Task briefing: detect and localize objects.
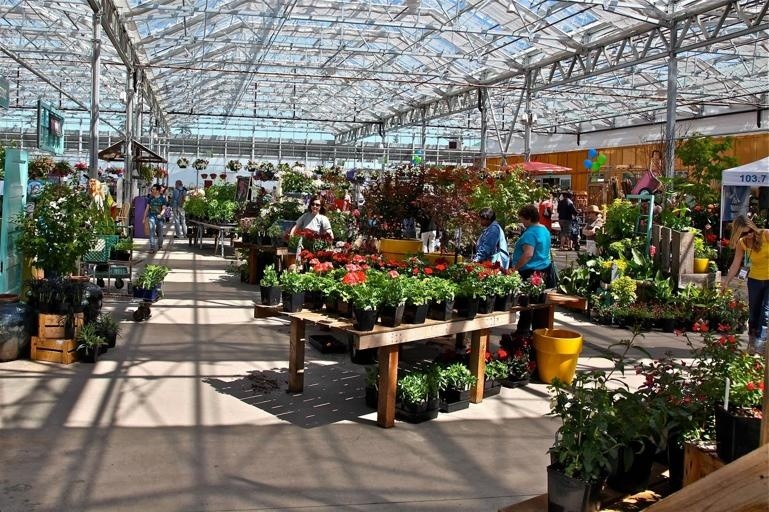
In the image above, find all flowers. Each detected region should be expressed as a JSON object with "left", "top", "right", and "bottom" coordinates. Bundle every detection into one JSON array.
[
  {"left": 261, "top": 250, "right": 547, "bottom": 285},
  {"left": 634, "top": 317, "right": 768, "bottom": 506},
  {"left": 496, "top": 338, "right": 537, "bottom": 380}
]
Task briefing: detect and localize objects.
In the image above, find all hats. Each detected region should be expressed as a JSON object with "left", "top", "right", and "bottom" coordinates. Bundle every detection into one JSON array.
[{"left": 585, "top": 205, "right": 601, "bottom": 215}]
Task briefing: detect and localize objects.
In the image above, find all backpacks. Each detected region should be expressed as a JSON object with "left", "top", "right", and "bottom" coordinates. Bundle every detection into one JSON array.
[{"left": 175, "top": 187, "right": 188, "bottom": 207}]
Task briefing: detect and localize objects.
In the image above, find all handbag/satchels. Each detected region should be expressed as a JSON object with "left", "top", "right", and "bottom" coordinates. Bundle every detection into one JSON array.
[
  {"left": 545, "top": 261, "right": 560, "bottom": 288},
  {"left": 544, "top": 206, "right": 552, "bottom": 218}
]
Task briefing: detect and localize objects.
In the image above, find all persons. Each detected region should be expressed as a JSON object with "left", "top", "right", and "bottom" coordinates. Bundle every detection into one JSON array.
[
  {"left": 511, "top": 204, "right": 552, "bottom": 337},
  {"left": 288, "top": 194, "right": 335, "bottom": 273},
  {"left": 719, "top": 215, "right": 769, "bottom": 355},
  {"left": 538, "top": 190, "right": 581, "bottom": 251},
  {"left": 582, "top": 205, "right": 603, "bottom": 257},
  {"left": 421, "top": 218, "right": 437, "bottom": 253},
  {"left": 473, "top": 207, "right": 510, "bottom": 270},
  {"left": 142, "top": 180, "right": 187, "bottom": 254},
  {"left": 342, "top": 188, "right": 417, "bottom": 248}
]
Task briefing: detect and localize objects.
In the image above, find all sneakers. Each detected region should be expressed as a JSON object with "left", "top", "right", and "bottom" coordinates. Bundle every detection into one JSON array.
[
  {"left": 149, "top": 246, "right": 164, "bottom": 254},
  {"left": 558, "top": 245, "right": 573, "bottom": 252},
  {"left": 173, "top": 234, "right": 187, "bottom": 240}
]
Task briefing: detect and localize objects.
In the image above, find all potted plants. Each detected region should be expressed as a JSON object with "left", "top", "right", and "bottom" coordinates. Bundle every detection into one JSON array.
[
  {"left": 363, "top": 365, "right": 379, "bottom": 409},
  {"left": 397, "top": 368, "right": 431, "bottom": 414},
  {"left": 8, "top": 155, "right": 166, "bottom": 359},
  {"left": 236, "top": 244, "right": 249, "bottom": 284},
  {"left": 492, "top": 359, "right": 510, "bottom": 387},
  {"left": 422, "top": 369, "right": 450, "bottom": 410},
  {"left": 443, "top": 361, "right": 479, "bottom": 401},
  {"left": 263, "top": 285, "right": 545, "bottom": 333},
  {"left": 484, "top": 361, "right": 498, "bottom": 390},
  {"left": 561, "top": 195, "right": 748, "bottom": 331},
  {"left": 177, "top": 152, "right": 548, "bottom": 236},
  {"left": 543, "top": 330, "right": 668, "bottom": 512}
]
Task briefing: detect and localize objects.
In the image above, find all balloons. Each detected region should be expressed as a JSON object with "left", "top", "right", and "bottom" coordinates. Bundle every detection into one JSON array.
[
  {"left": 591, "top": 161, "right": 600, "bottom": 172},
  {"left": 584, "top": 159, "right": 593, "bottom": 169},
  {"left": 597, "top": 154, "right": 607, "bottom": 165},
  {"left": 410, "top": 150, "right": 422, "bottom": 165},
  {"left": 588, "top": 148, "right": 597, "bottom": 158}
]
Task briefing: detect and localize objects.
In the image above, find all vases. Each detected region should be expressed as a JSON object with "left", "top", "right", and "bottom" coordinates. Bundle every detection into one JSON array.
[{"left": 501, "top": 376, "right": 530, "bottom": 389}]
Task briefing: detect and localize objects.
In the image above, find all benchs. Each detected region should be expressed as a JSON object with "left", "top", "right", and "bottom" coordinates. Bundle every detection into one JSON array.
[{"left": 275, "top": 300, "right": 537, "bottom": 394}]
